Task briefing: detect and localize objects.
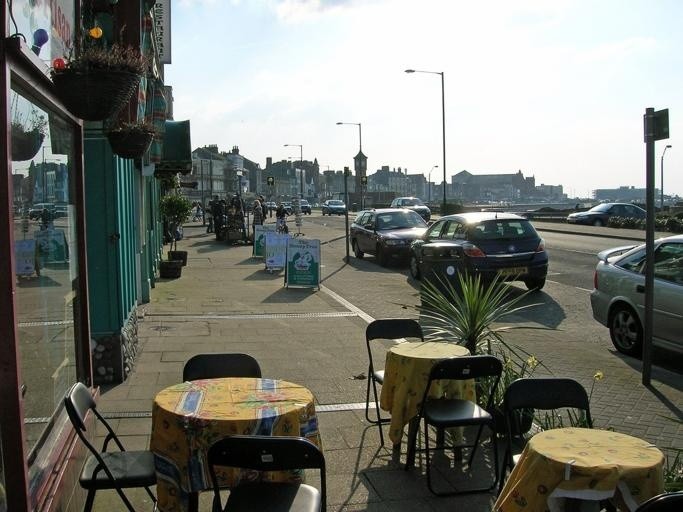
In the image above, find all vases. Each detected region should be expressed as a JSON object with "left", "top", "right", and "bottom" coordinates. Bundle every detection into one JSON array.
[{"left": 496, "top": 401, "right": 536, "bottom": 433}]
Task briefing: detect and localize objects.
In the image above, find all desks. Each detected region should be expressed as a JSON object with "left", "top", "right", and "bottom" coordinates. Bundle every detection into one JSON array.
[
  {"left": 150, "top": 376, "right": 323, "bottom": 511},
  {"left": 490, "top": 427, "right": 667, "bottom": 512},
  {"left": 380, "top": 341, "right": 478, "bottom": 471}
]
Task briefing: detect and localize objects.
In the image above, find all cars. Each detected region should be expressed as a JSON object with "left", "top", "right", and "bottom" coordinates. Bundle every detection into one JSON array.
[
  {"left": 588, "top": 233, "right": 683, "bottom": 357},
  {"left": 247, "top": 198, "right": 312, "bottom": 215},
  {"left": 347, "top": 195, "right": 550, "bottom": 295},
  {"left": 320, "top": 198, "right": 348, "bottom": 216},
  {"left": 565, "top": 202, "right": 647, "bottom": 227},
  {"left": 13, "top": 202, "right": 71, "bottom": 221}
]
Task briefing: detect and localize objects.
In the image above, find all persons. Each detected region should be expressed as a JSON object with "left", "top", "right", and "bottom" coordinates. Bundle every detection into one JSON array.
[
  {"left": 275, "top": 205, "right": 289, "bottom": 226},
  {"left": 191, "top": 193, "right": 266, "bottom": 237}
]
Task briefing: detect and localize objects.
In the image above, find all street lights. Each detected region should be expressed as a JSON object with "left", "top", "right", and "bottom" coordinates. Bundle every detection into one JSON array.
[
  {"left": 335, "top": 121, "right": 363, "bottom": 209},
  {"left": 15, "top": 145, "right": 61, "bottom": 202},
  {"left": 403, "top": 67, "right": 448, "bottom": 216},
  {"left": 428, "top": 165, "right": 438, "bottom": 206},
  {"left": 236, "top": 169, "right": 243, "bottom": 196},
  {"left": 288, "top": 156, "right": 301, "bottom": 159},
  {"left": 282, "top": 144, "right": 304, "bottom": 198},
  {"left": 659, "top": 144, "right": 673, "bottom": 218}
]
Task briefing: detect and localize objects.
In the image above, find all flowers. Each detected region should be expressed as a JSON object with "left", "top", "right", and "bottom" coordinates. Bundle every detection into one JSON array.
[{"left": 491, "top": 355, "right": 541, "bottom": 410}]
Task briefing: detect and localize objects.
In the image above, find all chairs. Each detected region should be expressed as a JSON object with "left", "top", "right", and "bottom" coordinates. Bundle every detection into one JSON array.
[
  {"left": 363, "top": 318, "right": 427, "bottom": 445},
  {"left": 403, "top": 355, "right": 504, "bottom": 498},
  {"left": 63, "top": 381, "right": 158, "bottom": 511},
  {"left": 205, "top": 435, "right": 327, "bottom": 511},
  {"left": 497, "top": 378, "right": 594, "bottom": 499},
  {"left": 634, "top": 489, "right": 683, "bottom": 512},
  {"left": 181, "top": 353, "right": 262, "bottom": 383}
]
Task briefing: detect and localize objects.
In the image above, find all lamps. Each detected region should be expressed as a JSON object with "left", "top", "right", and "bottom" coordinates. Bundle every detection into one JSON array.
[
  {"left": 31, "top": 29, "right": 49, "bottom": 57},
  {"left": 80, "top": 27, "right": 102, "bottom": 40}
]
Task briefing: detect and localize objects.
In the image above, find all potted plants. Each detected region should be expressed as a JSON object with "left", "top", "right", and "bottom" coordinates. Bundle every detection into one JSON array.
[
  {"left": 50, "top": 42, "right": 149, "bottom": 123},
  {"left": 156, "top": 195, "right": 192, "bottom": 279},
  {"left": 106, "top": 119, "right": 163, "bottom": 160},
  {"left": 10, "top": 92, "right": 46, "bottom": 162},
  {"left": 163, "top": 191, "right": 188, "bottom": 267}
]
